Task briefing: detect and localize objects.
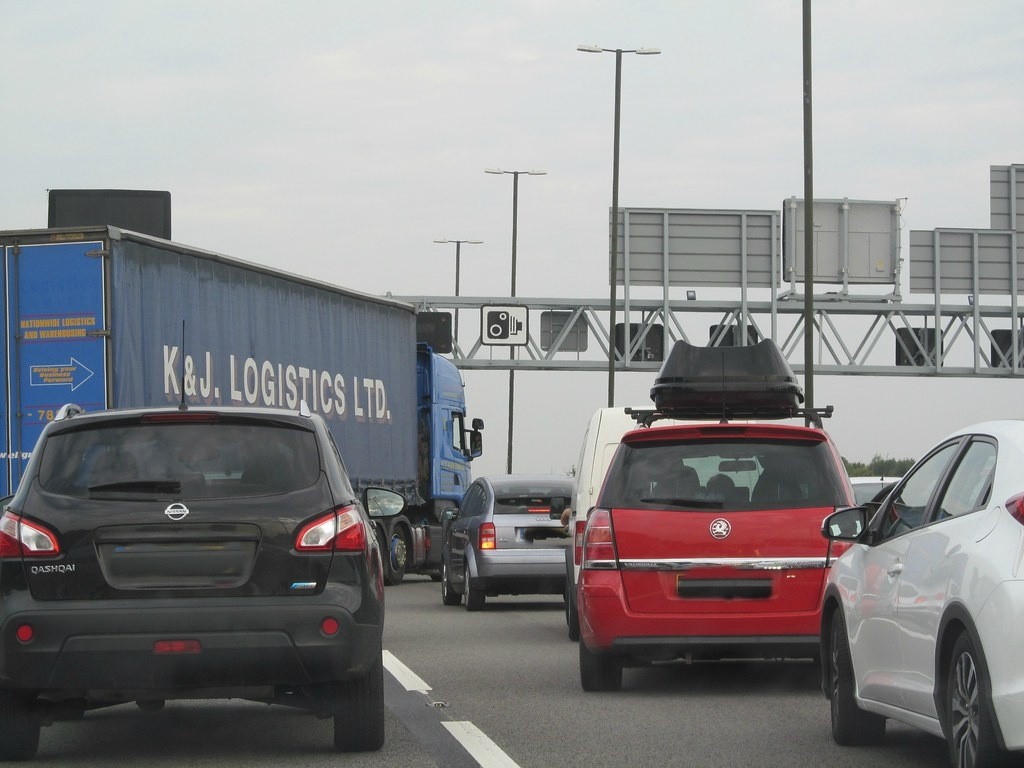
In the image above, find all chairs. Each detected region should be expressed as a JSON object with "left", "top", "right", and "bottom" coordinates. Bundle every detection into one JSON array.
[{"left": 649, "top": 466, "right": 820, "bottom": 505}]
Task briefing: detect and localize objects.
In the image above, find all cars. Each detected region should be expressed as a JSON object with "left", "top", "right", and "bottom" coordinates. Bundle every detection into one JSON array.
[
  {"left": 813, "top": 418, "right": 1024, "bottom": 768},
  {"left": 439, "top": 477, "right": 570, "bottom": 611}
]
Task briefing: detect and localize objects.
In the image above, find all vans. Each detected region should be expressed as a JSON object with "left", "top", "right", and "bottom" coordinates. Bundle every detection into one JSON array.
[{"left": 562, "top": 403, "right": 816, "bottom": 639}]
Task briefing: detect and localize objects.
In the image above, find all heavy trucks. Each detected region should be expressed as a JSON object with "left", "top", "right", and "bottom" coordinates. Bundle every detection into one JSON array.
[{"left": 0, "top": 225, "right": 488, "bottom": 587}]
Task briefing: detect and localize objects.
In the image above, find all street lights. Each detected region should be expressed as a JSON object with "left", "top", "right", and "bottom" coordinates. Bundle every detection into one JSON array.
[
  {"left": 578, "top": 46, "right": 663, "bottom": 409},
  {"left": 483, "top": 167, "right": 550, "bottom": 476},
  {"left": 434, "top": 238, "right": 485, "bottom": 358}
]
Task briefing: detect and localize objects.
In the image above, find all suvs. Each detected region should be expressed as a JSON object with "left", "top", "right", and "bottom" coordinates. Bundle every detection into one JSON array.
[
  {"left": 579, "top": 338, "right": 859, "bottom": 694},
  {"left": 0, "top": 385, "right": 409, "bottom": 760}
]
